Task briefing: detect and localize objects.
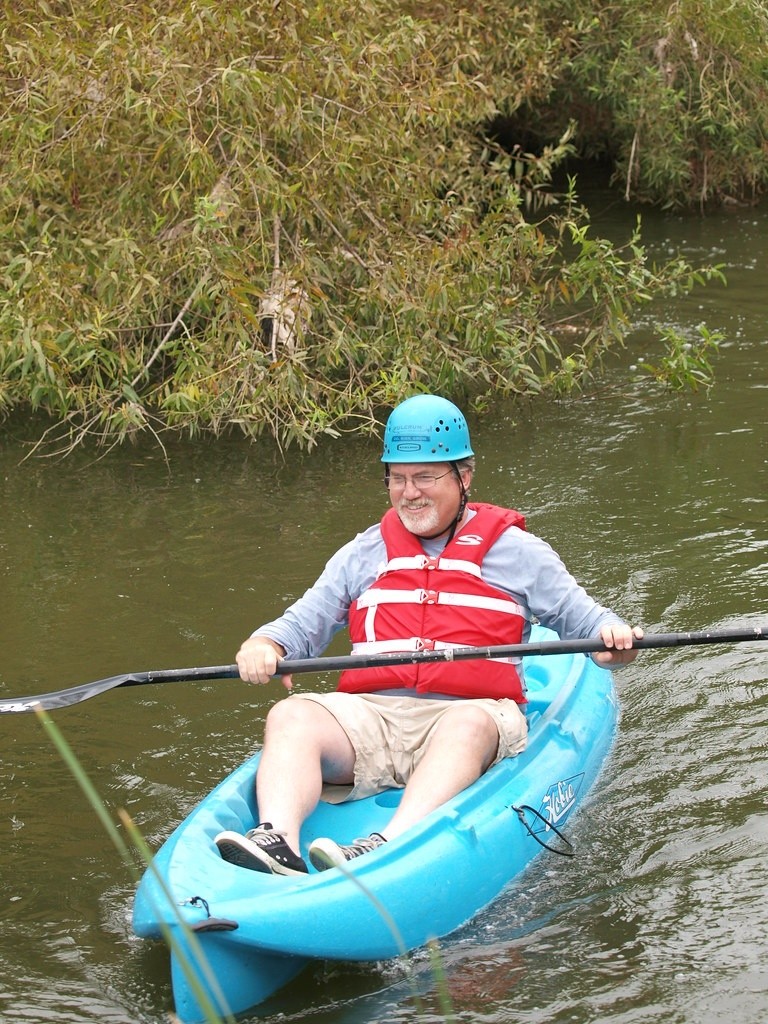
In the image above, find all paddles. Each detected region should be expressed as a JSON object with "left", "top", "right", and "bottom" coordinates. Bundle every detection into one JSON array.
[{"left": 0, "top": 623, "right": 768, "bottom": 714}]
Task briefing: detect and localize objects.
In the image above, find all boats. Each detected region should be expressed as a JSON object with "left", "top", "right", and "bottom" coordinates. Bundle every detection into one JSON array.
[{"left": 130, "top": 622, "right": 622, "bottom": 1024}]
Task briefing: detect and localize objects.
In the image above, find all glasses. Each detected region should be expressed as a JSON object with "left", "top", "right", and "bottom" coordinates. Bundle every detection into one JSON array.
[{"left": 383, "top": 468, "right": 454, "bottom": 489}]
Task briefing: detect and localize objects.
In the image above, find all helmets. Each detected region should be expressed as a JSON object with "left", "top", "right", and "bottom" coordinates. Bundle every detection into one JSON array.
[{"left": 380, "top": 394, "right": 475, "bottom": 462}]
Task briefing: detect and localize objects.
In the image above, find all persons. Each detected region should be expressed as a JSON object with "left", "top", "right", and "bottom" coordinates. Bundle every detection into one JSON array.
[{"left": 214, "top": 395, "right": 644, "bottom": 878}]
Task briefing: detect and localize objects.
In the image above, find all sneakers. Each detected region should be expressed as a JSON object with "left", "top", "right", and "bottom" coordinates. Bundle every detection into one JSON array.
[
  {"left": 213, "top": 823, "right": 308, "bottom": 876},
  {"left": 309, "top": 832, "right": 388, "bottom": 872}
]
